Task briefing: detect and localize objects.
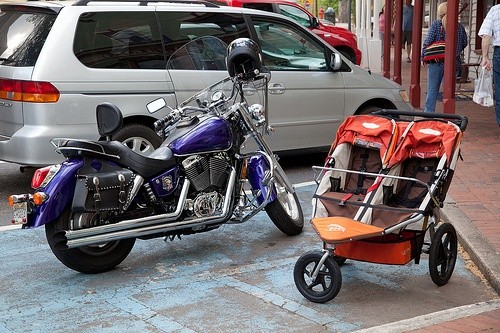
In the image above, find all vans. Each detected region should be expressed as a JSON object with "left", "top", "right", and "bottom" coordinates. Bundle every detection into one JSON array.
[{"left": 0, "top": 0, "right": 415, "bottom": 168}]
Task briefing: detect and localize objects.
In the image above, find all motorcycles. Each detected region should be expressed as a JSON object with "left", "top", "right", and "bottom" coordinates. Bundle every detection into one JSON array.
[{"left": 8, "top": 34, "right": 305, "bottom": 275}]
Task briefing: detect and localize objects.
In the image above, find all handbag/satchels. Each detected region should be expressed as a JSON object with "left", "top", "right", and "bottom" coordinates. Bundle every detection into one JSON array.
[{"left": 423, "top": 20, "right": 446, "bottom": 64}]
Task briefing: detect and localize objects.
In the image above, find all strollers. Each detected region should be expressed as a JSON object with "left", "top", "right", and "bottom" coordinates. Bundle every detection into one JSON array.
[{"left": 293, "top": 109, "right": 468, "bottom": 304}]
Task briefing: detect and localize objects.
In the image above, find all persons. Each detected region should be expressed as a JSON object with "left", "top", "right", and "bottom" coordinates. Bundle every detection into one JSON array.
[
  {"left": 378, "top": 0, "right": 416, "bottom": 62},
  {"left": 421, "top": 2, "right": 467, "bottom": 118},
  {"left": 477, "top": 4, "right": 500, "bottom": 128}
]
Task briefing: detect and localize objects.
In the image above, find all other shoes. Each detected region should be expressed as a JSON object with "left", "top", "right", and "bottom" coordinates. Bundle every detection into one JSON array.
[{"left": 407, "top": 57, "right": 411, "bottom": 63}]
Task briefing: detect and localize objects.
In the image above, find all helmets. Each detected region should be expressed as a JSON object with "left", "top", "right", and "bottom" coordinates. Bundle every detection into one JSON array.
[{"left": 225, "top": 37, "right": 262, "bottom": 80}]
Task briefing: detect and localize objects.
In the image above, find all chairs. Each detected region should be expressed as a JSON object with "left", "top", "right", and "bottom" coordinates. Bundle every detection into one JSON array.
[{"left": 456, "top": 49, "right": 483, "bottom": 87}]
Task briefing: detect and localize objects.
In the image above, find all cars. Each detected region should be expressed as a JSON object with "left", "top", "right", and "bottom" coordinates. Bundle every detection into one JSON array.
[{"left": 207, "top": 0, "right": 362, "bottom": 71}]
